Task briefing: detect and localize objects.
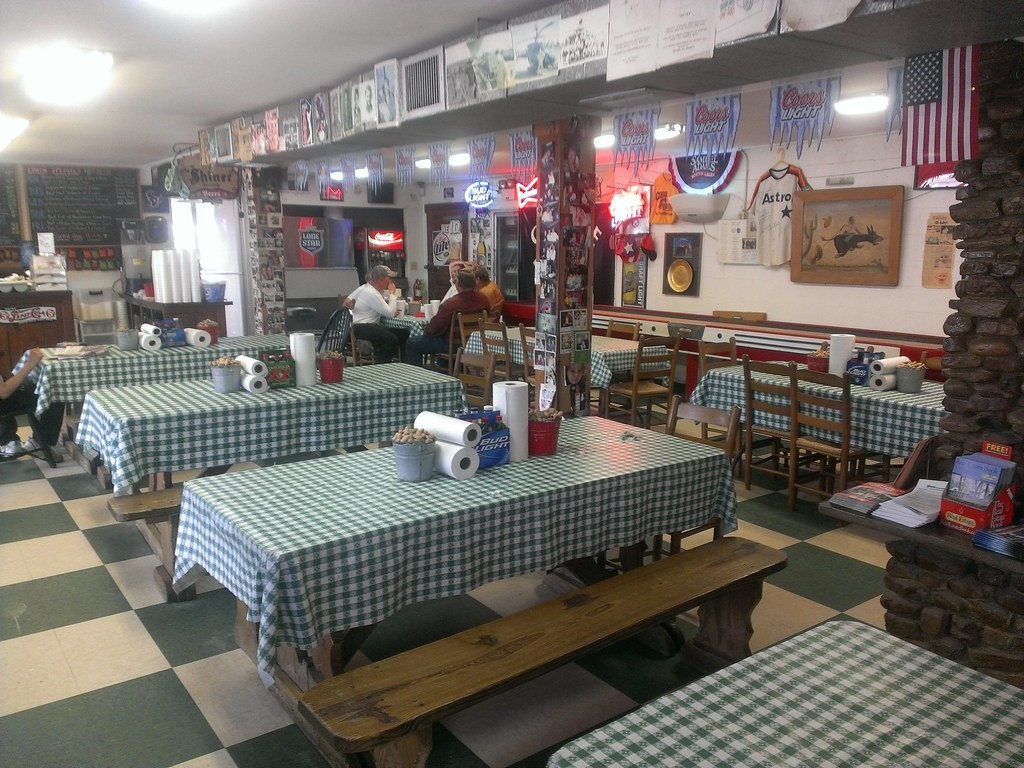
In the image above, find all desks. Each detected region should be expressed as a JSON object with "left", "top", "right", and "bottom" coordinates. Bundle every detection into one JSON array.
[
  {"left": 11, "top": 331, "right": 326, "bottom": 454},
  {"left": 374, "top": 311, "right": 440, "bottom": 368},
  {"left": 170, "top": 416, "right": 738, "bottom": 701},
  {"left": 549, "top": 611, "right": 1024, "bottom": 768},
  {"left": 691, "top": 358, "right": 950, "bottom": 484},
  {"left": 463, "top": 326, "right": 671, "bottom": 425},
  {"left": 118, "top": 292, "right": 233, "bottom": 338},
  {"left": 73, "top": 361, "right": 472, "bottom": 542}
]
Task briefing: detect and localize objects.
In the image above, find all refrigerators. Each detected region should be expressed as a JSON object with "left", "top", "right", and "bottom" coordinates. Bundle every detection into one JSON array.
[{"left": 493, "top": 208, "right": 536, "bottom": 301}]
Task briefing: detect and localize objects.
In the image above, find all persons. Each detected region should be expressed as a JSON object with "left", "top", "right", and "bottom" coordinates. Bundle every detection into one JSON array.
[
  {"left": 343, "top": 78, "right": 395, "bottom": 130},
  {"left": 403, "top": 267, "right": 490, "bottom": 368},
  {"left": 495, "top": 49, "right": 510, "bottom": 88},
  {"left": 536, "top": 47, "right": 545, "bottom": 75},
  {"left": 0, "top": 347, "right": 65, "bottom": 463},
  {"left": 441, "top": 261, "right": 505, "bottom": 343},
  {"left": 342, "top": 265, "right": 410, "bottom": 364}
]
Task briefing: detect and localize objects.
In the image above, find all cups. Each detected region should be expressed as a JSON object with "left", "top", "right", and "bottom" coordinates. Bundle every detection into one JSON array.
[
  {"left": 143, "top": 282, "right": 155, "bottom": 298},
  {"left": 424, "top": 304, "right": 432, "bottom": 317},
  {"left": 151, "top": 249, "right": 201, "bottom": 302},
  {"left": 447, "top": 233, "right": 462, "bottom": 264},
  {"left": 116, "top": 299, "right": 129, "bottom": 330},
  {"left": 397, "top": 300, "right": 405, "bottom": 315},
  {"left": 430, "top": 300, "right": 440, "bottom": 316}
]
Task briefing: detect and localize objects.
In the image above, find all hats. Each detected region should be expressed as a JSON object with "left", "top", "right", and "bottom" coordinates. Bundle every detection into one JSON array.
[
  {"left": 457, "top": 269, "right": 476, "bottom": 281},
  {"left": 371, "top": 266, "right": 398, "bottom": 282}
]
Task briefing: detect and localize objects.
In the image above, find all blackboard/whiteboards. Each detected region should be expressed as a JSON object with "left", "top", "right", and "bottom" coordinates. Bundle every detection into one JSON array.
[
  {"left": 0, "top": 163, "right": 22, "bottom": 249},
  {"left": 21, "top": 163, "right": 144, "bottom": 249}
]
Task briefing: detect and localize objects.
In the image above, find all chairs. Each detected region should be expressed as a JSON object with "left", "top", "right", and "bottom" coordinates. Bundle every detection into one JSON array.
[{"left": 316, "top": 291, "right": 918, "bottom": 511}]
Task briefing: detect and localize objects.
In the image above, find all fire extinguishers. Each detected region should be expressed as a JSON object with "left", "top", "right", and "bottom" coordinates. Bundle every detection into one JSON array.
[{"left": 413, "top": 278, "right": 423, "bottom": 301}]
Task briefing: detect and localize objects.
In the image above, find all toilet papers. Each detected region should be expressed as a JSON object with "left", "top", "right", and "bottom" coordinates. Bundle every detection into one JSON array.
[
  {"left": 829, "top": 333, "right": 856, "bottom": 377},
  {"left": 289, "top": 333, "right": 316, "bottom": 386},
  {"left": 869, "top": 355, "right": 912, "bottom": 391},
  {"left": 414, "top": 410, "right": 482, "bottom": 480},
  {"left": 139, "top": 324, "right": 161, "bottom": 352},
  {"left": 235, "top": 355, "right": 270, "bottom": 393},
  {"left": 184, "top": 328, "right": 211, "bottom": 348},
  {"left": 492, "top": 381, "right": 528, "bottom": 461}
]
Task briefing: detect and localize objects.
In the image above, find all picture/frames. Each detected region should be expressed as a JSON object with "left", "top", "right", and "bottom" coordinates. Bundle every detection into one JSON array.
[{"left": 790, "top": 187, "right": 903, "bottom": 286}]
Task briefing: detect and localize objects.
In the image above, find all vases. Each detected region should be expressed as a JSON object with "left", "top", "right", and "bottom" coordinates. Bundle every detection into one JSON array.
[{"left": 407, "top": 300, "right": 423, "bottom": 316}]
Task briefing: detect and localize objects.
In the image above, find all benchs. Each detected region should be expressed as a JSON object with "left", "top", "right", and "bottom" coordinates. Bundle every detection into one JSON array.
[
  {"left": 296, "top": 530, "right": 792, "bottom": 767},
  {"left": 105, "top": 480, "right": 218, "bottom": 600}
]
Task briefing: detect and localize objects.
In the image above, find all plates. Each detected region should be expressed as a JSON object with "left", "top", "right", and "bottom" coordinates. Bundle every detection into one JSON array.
[{"left": 668, "top": 259, "right": 693, "bottom": 292}]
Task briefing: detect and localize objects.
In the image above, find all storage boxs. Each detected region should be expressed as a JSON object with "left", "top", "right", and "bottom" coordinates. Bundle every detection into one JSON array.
[
  {"left": 256, "top": 349, "right": 297, "bottom": 388},
  {"left": 453, "top": 404, "right": 511, "bottom": 468},
  {"left": 152, "top": 319, "right": 187, "bottom": 347}
]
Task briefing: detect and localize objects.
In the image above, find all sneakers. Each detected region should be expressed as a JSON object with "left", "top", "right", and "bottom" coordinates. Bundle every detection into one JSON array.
[
  {"left": 0, "top": 440, "right": 25, "bottom": 457},
  {"left": 22, "top": 437, "right": 63, "bottom": 463}
]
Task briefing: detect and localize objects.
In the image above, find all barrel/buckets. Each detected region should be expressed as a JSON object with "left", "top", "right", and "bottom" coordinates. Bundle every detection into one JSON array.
[
  {"left": 528, "top": 416, "right": 561, "bottom": 456},
  {"left": 319, "top": 356, "right": 344, "bottom": 383},
  {"left": 197, "top": 323, "right": 218, "bottom": 344},
  {"left": 392, "top": 436, "right": 436, "bottom": 481},
  {"left": 117, "top": 329, "right": 138, "bottom": 350},
  {"left": 201, "top": 282, "right": 225, "bottom": 302},
  {"left": 897, "top": 365, "right": 925, "bottom": 393},
  {"left": 807, "top": 353, "right": 829, "bottom": 374},
  {"left": 408, "top": 303, "right": 421, "bottom": 315},
  {"left": 210, "top": 363, "right": 240, "bottom": 393}
]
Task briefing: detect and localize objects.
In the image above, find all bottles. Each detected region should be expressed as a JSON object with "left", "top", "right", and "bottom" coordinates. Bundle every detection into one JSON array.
[
  {"left": 481, "top": 418, "right": 492, "bottom": 436},
  {"left": 471, "top": 407, "right": 481, "bottom": 426},
  {"left": 484, "top": 405, "right": 493, "bottom": 413},
  {"left": 851, "top": 347, "right": 880, "bottom": 365},
  {"left": 492, "top": 416, "right": 506, "bottom": 431},
  {"left": 262, "top": 352, "right": 291, "bottom": 367},
  {"left": 477, "top": 236, "right": 486, "bottom": 269},
  {"left": 455, "top": 408, "right": 462, "bottom": 419}
]
